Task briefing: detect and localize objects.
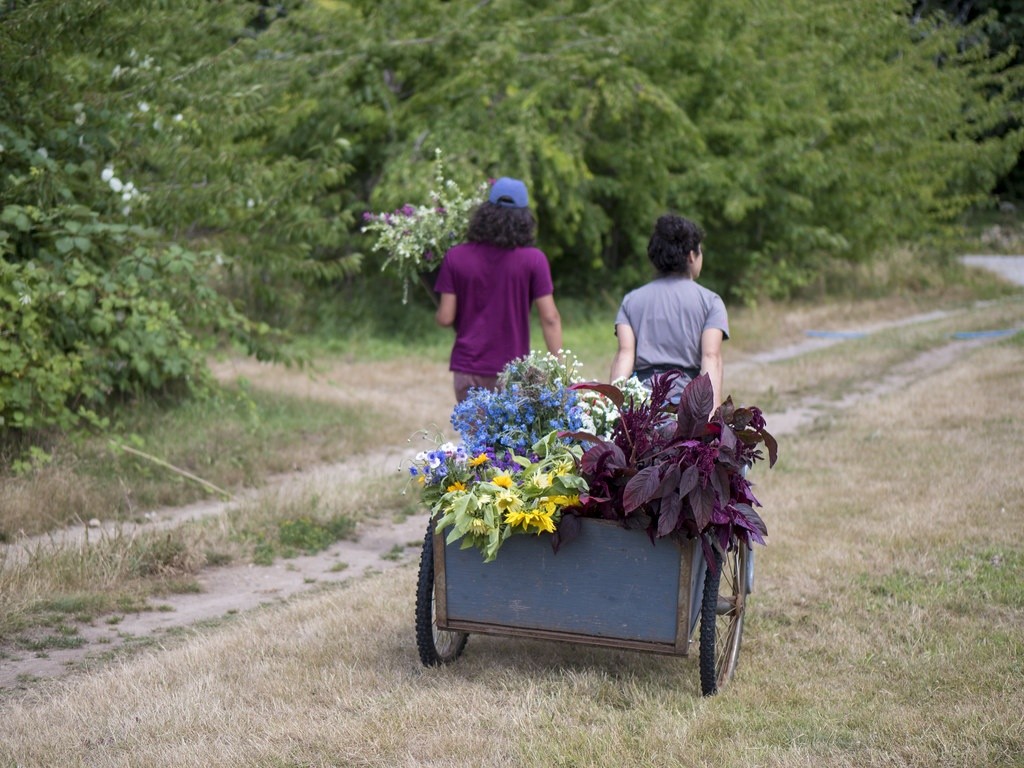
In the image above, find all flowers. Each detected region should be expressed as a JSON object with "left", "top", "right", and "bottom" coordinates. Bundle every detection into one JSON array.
[
  {"left": 361, "top": 148, "right": 495, "bottom": 310},
  {"left": 397, "top": 347, "right": 778, "bottom": 575}
]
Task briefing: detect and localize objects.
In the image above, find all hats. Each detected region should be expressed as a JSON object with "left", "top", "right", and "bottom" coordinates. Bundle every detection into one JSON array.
[{"left": 488, "top": 177, "right": 529, "bottom": 208}]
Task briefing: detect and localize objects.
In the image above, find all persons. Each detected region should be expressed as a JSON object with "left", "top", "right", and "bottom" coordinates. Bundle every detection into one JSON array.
[
  {"left": 609, "top": 213, "right": 730, "bottom": 431},
  {"left": 434, "top": 177, "right": 564, "bottom": 405}
]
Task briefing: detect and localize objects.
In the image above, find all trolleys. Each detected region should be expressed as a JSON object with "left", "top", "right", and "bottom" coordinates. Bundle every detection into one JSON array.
[{"left": 412, "top": 468, "right": 757, "bottom": 697}]
